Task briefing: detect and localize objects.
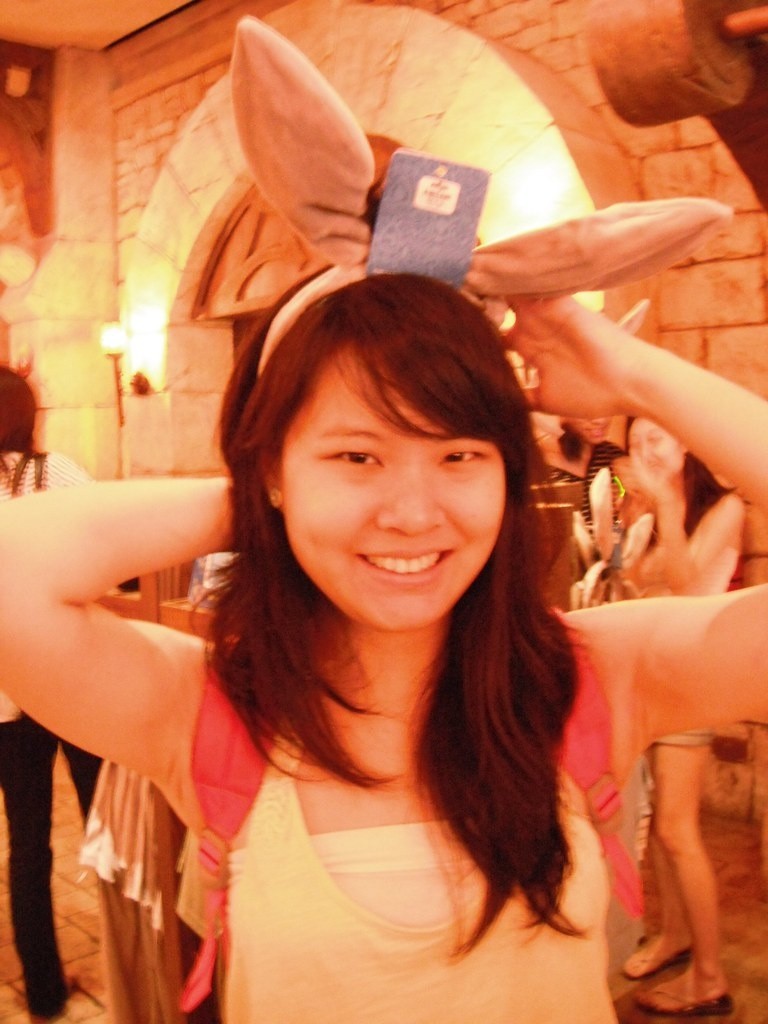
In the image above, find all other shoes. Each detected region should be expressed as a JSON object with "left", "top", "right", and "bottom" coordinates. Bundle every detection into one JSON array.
[{"left": 28, "top": 974, "right": 79, "bottom": 1024}]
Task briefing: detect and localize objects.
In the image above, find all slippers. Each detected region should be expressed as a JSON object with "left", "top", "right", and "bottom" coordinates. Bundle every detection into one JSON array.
[
  {"left": 632, "top": 979, "right": 734, "bottom": 1017},
  {"left": 622, "top": 933, "right": 694, "bottom": 979}
]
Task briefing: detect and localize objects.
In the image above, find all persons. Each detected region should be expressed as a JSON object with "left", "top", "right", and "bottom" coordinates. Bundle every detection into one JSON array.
[
  {"left": 538, "top": 405, "right": 747, "bottom": 1015},
  {"left": 0, "top": 364, "right": 104, "bottom": 1024},
  {"left": 0, "top": 270, "right": 768, "bottom": 1024}
]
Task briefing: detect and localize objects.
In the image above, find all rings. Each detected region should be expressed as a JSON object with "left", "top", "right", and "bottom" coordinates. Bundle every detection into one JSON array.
[{"left": 499, "top": 309, "right": 517, "bottom": 333}]
